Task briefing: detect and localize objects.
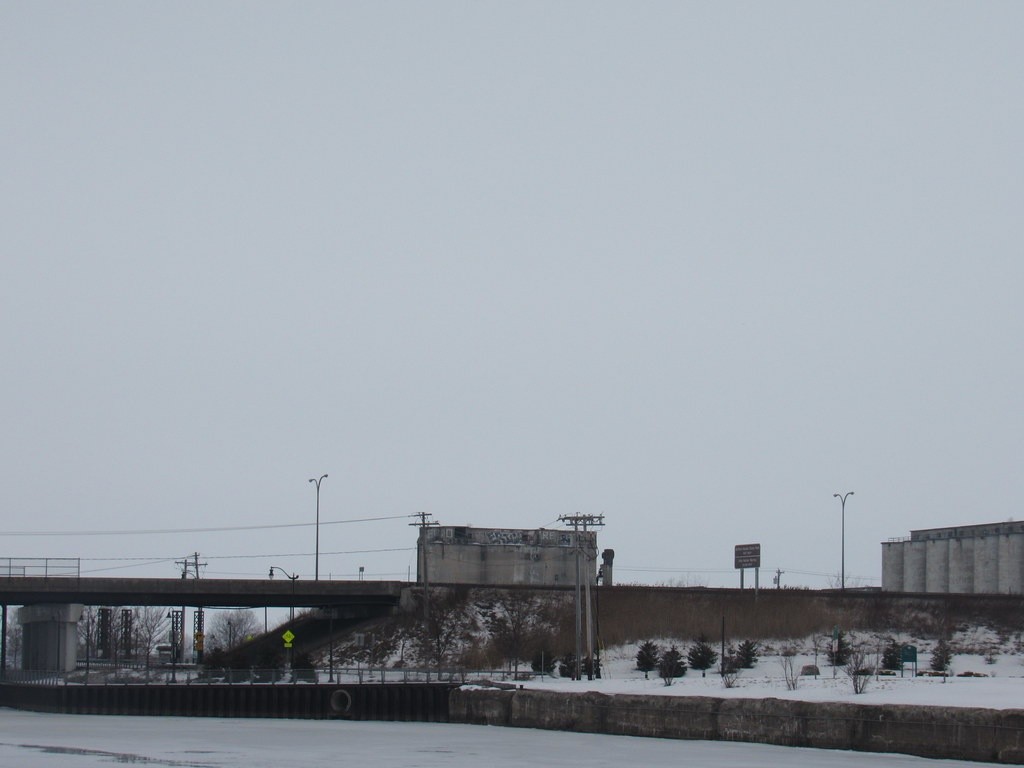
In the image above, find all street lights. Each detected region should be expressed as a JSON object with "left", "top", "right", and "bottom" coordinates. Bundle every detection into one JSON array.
[
  {"left": 833, "top": 492, "right": 854, "bottom": 588},
  {"left": 269, "top": 566, "right": 299, "bottom": 679},
  {"left": 308, "top": 475, "right": 329, "bottom": 579}
]
